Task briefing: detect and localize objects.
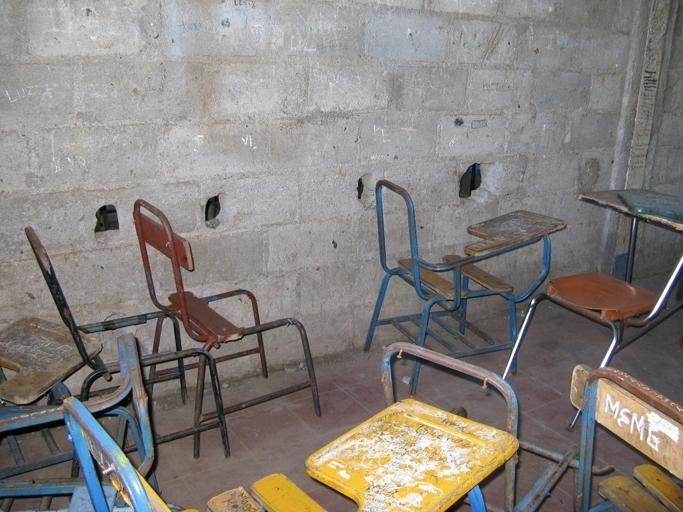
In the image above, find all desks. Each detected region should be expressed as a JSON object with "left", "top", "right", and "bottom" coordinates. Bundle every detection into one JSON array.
[{"left": 578, "top": 188, "right": 683, "bottom": 343}]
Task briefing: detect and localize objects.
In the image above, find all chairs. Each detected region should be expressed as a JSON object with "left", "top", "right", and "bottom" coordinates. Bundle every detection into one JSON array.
[
  {"left": 133, "top": 199, "right": 322, "bottom": 457},
  {"left": 580, "top": 368, "right": 683, "bottom": 510},
  {"left": 1, "top": 334, "right": 184, "bottom": 511},
  {"left": 501, "top": 256, "right": 683, "bottom": 429},
  {"left": 381, "top": 343, "right": 614, "bottom": 511},
  {"left": 0, "top": 317, "right": 103, "bottom": 460},
  {"left": 61, "top": 396, "right": 520, "bottom": 511},
  {"left": 364, "top": 181, "right": 567, "bottom": 396},
  {"left": 25, "top": 225, "right": 230, "bottom": 502}
]
